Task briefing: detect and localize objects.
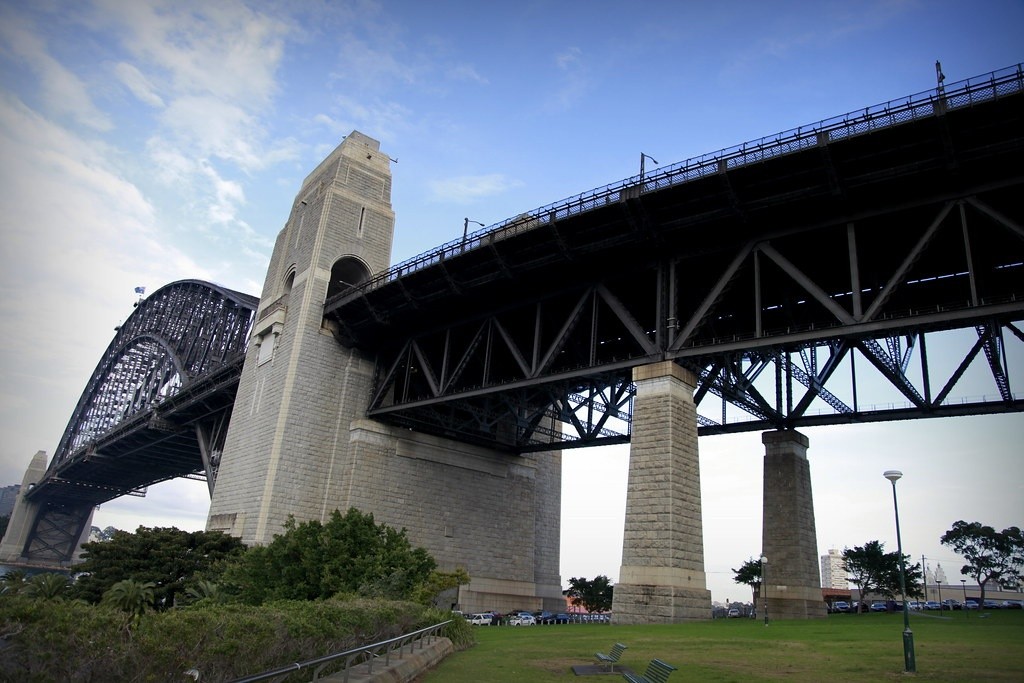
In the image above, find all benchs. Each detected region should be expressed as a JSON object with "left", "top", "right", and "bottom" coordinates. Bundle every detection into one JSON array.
[
  {"left": 594, "top": 642, "right": 628, "bottom": 672},
  {"left": 623, "top": 658, "right": 679, "bottom": 683}
]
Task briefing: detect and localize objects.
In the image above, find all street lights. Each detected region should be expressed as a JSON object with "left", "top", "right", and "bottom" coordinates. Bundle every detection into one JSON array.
[
  {"left": 933, "top": 60, "right": 949, "bottom": 112},
  {"left": 760, "top": 556, "right": 769, "bottom": 626},
  {"left": 883, "top": 469, "right": 916, "bottom": 672},
  {"left": 936, "top": 580, "right": 943, "bottom": 616},
  {"left": 460, "top": 216, "right": 485, "bottom": 252},
  {"left": 639, "top": 151, "right": 659, "bottom": 194},
  {"left": 960, "top": 579, "right": 969, "bottom": 616}
]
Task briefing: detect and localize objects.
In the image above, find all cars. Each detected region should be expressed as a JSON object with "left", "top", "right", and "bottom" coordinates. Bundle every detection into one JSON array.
[
  {"left": 451, "top": 609, "right": 610, "bottom": 626},
  {"left": 749, "top": 609, "right": 757, "bottom": 618},
  {"left": 727, "top": 609, "right": 741, "bottom": 618},
  {"left": 826, "top": 599, "right": 1024, "bottom": 614}
]
